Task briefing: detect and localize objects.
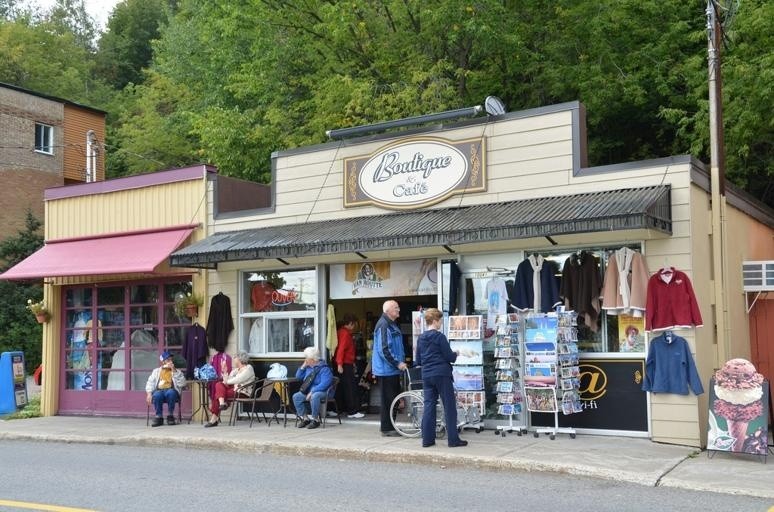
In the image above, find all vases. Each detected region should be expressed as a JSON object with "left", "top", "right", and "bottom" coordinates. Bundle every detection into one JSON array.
[
  {"left": 184, "top": 305, "right": 198, "bottom": 317},
  {"left": 35, "top": 314, "right": 48, "bottom": 324}
]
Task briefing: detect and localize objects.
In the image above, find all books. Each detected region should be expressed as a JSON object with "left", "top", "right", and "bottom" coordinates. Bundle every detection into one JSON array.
[
  {"left": 409, "top": 311, "right": 487, "bottom": 416},
  {"left": 494, "top": 310, "right": 584, "bottom": 416}
]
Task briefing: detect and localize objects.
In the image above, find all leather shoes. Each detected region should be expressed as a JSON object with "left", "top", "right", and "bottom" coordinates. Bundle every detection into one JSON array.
[
  {"left": 202, "top": 421, "right": 218, "bottom": 427},
  {"left": 150, "top": 415, "right": 177, "bottom": 427},
  {"left": 218, "top": 403, "right": 228, "bottom": 411}
]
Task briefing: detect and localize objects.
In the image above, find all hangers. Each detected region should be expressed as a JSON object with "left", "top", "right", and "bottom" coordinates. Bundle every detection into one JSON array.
[
  {"left": 576, "top": 247, "right": 582, "bottom": 257},
  {"left": 295, "top": 377, "right": 341, "bottom": 428},
  {"left": 621, "top": 239, "right": 632, "bottom": 257},
  {"left": 192, "top": 319, "right": 201, "bottom": 328},
  {"left": 532, "top": 249, "right": 539, "bottom": 258},
  {"left": 491, "top": 272, "right": 500, "bottom": 282},
  {"left": 660, "top": 257, "right": 674, "bottom": 274},
  {"left": 233, "top": 377, "right": 280, "bottom": 428},
  {"left": 217, "top": 288, "right": 224, "bottom": 296},
  {"left": 664, "top": 329, "right": 673, "bottom": 338}
]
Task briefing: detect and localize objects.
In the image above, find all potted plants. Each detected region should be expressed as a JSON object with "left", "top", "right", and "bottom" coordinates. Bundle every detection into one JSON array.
[{"left": 174, "top": 291, "right": 204, "bottom": 318}]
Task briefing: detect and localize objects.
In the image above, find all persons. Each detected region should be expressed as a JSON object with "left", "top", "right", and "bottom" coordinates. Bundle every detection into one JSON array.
[
  {"left": 621, "top": 325, "right": 638, "bottom": 352},
  {"left": 358, "top": 263, "right": 377, "bottom": 281},
  {"left": 292, "top": 347, "right": 335, "bottom": 429},
  {"left": 371, "top": 299, "right": 408, "bottom": 437},
  {"left": 204, "top": 350, "right": 256, "bottom": 427},
  {"left": 145, "top": 352, "right": 187, "bottom": 428},
  {"left": 415, "top": 308, "right": 468, "bottom": 448},
  {"left": 335, "top": 312, "right": 366, "bottom": 418}
]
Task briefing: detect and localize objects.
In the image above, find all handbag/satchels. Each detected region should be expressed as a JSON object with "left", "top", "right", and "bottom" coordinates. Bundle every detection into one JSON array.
[{"left": 300, "top": 372, "right": 315, "bottom": 395}]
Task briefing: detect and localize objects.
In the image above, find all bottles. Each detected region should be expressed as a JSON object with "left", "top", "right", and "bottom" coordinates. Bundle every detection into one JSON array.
[{"left": 409, "top": 259, "right": 431, "bottom": 294}]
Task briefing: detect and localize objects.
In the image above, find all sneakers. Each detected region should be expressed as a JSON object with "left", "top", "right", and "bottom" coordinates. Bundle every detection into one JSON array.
[
  {"left": 448, "top": 438, "right": 468, "bottom": 448},
  {"left": 423, "top": 440, "right": 436, "bottom": 447},
  {"left": 326, "top": 409, "right": 339, "bottom": 419},
  {"left": 346, "top": 411, "right": 364, "bottom": 419},
  {"left": 382, "top": 429, "right": 403, "bottom": 438},
  {"left": 299, "top": 418, "right": 320, "bottom": 428}
]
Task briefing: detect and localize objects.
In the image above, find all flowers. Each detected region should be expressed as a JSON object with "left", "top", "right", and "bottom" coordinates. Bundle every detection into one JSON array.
[{"left": 25, "top": 299, "right": 49, "bottom": 317}]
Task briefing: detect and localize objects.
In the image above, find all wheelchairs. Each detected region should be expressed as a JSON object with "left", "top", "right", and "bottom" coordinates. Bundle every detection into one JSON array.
[{"left": 389, "top": 360, "right": 480, "bottom": 443}]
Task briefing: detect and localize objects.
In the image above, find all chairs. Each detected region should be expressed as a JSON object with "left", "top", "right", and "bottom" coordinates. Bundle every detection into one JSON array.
[
  {"left": 146, "top": 394, "right": 181, "bottom": 426},
  {"left": 218, "top": 377, "right": 260, "bottom": 426}
]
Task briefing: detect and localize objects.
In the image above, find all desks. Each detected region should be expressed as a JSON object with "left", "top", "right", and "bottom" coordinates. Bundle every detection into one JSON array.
[
  {"left": 266, "top": 377, "right": 303, "bottom": 428},
  {"left": 183, "top": 378, "right": 221, "bottom": 425}
]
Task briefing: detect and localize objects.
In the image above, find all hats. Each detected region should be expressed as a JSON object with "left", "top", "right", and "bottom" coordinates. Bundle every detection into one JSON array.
[{"left": 158, "top": 351, "right": 174, "bottom": 363}]
